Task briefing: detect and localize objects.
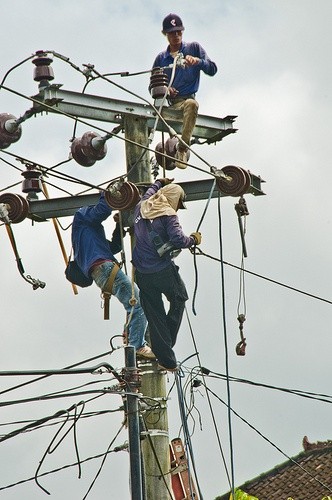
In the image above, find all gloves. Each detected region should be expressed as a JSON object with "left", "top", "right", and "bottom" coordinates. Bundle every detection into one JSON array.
[
  {"left": 155, "top": 178, "right": 174, "bottom": 187},
  {"left": 190, "top": 232, "right": 202, "bottom": 245},
  {"left": 113, "top": 213, "right": 120, "bottom": 223}
]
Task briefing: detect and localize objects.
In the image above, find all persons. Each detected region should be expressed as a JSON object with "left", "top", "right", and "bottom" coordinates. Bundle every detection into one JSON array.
[
  {"left": 72, "top": 190, "right": 156, "bottom": 358},
  {"left": 148, "top": 14, "right": 217, "bottom": 169},
  {"left": 132, "top": 178, "right": 202, "bottom": 370}
]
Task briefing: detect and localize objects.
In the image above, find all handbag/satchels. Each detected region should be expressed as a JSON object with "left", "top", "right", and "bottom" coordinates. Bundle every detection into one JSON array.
[{"left": 66, "top": 261, "right": 93, "bottom": 288}]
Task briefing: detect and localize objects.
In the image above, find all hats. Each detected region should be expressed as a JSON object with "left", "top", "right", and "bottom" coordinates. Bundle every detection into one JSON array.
[{"left": 162, "top": 13, "right": 185, "bottom": 34}]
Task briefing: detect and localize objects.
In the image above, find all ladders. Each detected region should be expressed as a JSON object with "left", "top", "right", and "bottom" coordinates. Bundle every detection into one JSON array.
[{"left": 169, "top": 437, "right": 199, "bottom": 500}]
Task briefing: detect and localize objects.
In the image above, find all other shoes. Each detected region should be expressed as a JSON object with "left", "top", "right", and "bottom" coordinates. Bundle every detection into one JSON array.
[
  {"left": 157, "top": 362, "right": 178, "bottom": 372},
  {"left": 175, "top": 142, "right": 188, "bottom": 169},
  {"left": 136, "top": 345, "right": 156, "bottom": 359}
]
